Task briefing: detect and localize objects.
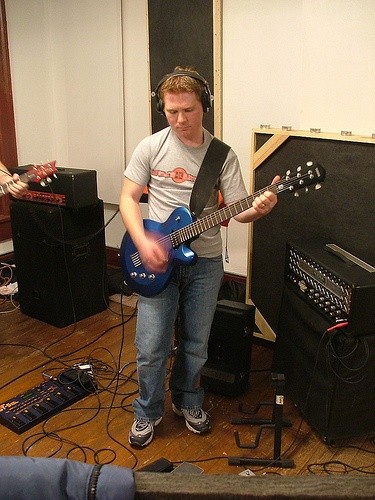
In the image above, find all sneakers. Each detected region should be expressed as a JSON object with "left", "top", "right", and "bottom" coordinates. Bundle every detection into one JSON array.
[
  {"left": 172, "top": 403, "right": 211, "bottom": 434},
  {"left": 127, "top": 415, "right": 163, "bottom": 449}
]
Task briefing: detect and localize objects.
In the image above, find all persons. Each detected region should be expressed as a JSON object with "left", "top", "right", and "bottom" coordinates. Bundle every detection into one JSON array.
[
  {"left": 0, "top": 161, "right": 29, "bottom": 199},
  {"left": 119, "top": 66, "right": 280, "bottom": 446}
]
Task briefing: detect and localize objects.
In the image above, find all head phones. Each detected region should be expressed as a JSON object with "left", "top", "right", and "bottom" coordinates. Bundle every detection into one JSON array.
[{"left": 154, "top": 70, "right": 214, "bottom": 116}]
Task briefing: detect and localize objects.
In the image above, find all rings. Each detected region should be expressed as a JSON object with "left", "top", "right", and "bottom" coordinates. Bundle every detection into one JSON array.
[{"left": 260, "top": 205, "right": 264, "bottom": 209}]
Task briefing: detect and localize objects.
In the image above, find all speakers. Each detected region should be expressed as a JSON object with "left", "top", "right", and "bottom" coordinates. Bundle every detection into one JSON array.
[
  {"left": 198, "top": 297, "right": 255, "bottom": 395},
  {"left": 268, "top": 283, "right": 375, "bottom": 447},
  {"left": 9, "top": 199, "right": 109, "bottom": 329}
]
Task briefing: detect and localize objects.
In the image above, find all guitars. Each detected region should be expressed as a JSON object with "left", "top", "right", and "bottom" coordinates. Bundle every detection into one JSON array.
[
  {"left": 0, "top": 159, "right": 60, "bottom": 199},
  {"left": 118, "top": 159, "right": 327, "bottom": 298}
]
